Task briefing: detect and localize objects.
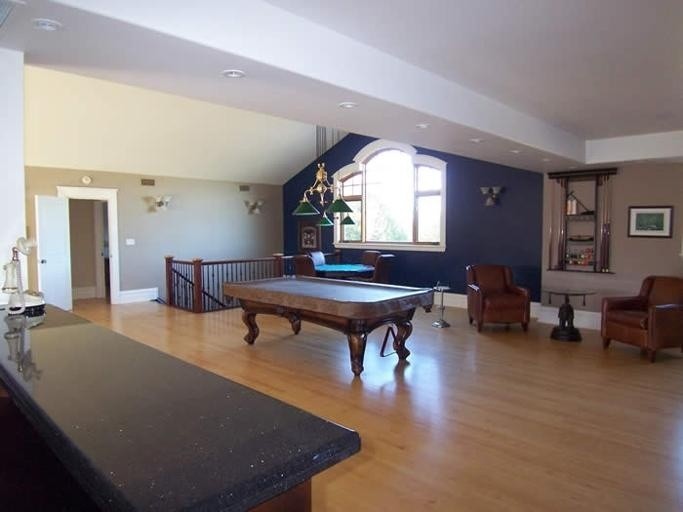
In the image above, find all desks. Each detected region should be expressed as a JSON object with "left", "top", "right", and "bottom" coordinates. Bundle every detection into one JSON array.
[{"left": 223, "top": 274, "right": 434, "bottom": 375}]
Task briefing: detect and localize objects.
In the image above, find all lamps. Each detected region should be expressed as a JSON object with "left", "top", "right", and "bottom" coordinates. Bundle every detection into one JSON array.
[
  {"left": 3, "top": 316, "right": 43, "bottom": 382},
  {"left": 2, "top": 237, "right": 47, "bottom": 328},
  {"left": 480, "top": 185, "right": 502, "bottom": 207},
  {"left": 246, "top": 200, "right": 263, "bottom": 215},
  {"left": 152, "top": 195, "right": 172, "bottom": 211},
  {"left": 292, "top": 122, "right": 355, "bottom": 227}
]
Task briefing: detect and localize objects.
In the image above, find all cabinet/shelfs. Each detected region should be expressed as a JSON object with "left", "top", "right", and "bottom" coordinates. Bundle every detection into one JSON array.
[{"left": 546, "top": 167, "right": 617, "bottom": 273}]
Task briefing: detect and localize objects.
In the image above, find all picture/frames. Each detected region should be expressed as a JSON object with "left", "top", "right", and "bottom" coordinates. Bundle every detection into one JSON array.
[
  {"left": 627, "top": 206, "right": 673, "bottom": 238},
  {"left": 297, "top": 220, "right": 322, "bottom": 254}
]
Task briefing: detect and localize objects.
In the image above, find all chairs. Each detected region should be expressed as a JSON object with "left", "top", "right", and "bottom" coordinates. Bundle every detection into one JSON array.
[
  {"left": 293, "top": 251, "right": 396, "bottom": 283},
  {"left": 466, "top": 264, "right": 530, "bottom": 333},
  {"left": 601, "top": 276, "right": 683, "bottom": 364}
]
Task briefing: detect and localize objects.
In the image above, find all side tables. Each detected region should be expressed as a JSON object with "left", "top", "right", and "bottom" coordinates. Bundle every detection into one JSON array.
[{"left": 541, "top": 288, "right": 598, "bottom": 343}]
